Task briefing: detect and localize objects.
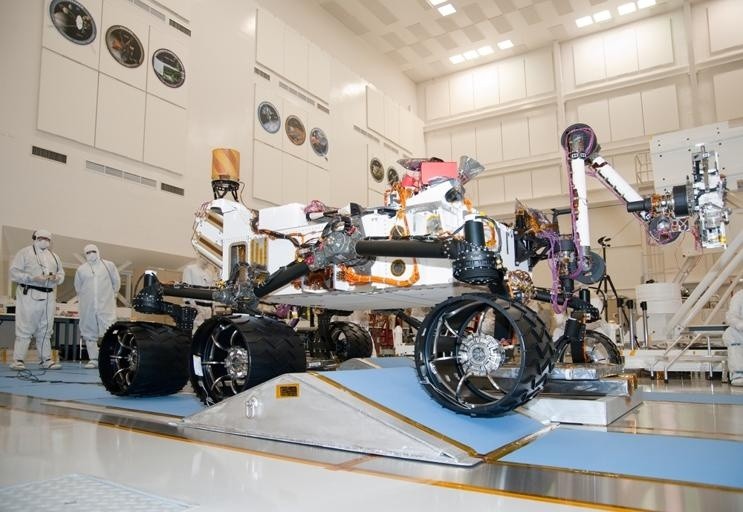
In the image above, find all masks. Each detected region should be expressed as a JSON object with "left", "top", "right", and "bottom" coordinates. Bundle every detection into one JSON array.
[
  {"left": 36, "top": 240, "right": 49, "bottom": 249},
  {"left": 87, "top": 254, "right": 98, "bottom": 262}
]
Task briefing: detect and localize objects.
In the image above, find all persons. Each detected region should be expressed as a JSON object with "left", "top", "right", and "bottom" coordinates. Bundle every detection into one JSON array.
[
  {"left": 722, "top": 288, "right": 743, "bottom": 386},
  {"left": 10, "top": 230, "right": 65, "bottom": 371},
  {"left": 73, "top": 244, "right": 122, "bottom": 368}
]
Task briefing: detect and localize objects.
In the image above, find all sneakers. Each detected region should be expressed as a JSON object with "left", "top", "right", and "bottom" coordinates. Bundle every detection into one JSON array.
[
  {"left": 39, "top": 361, "right": 62, "bottom": 370},
  {"left": 12, "top": 360, "right": 25, "bottom": 371},
  {"left": 84, "top": 362, "right": 98, "bottom": 368}
]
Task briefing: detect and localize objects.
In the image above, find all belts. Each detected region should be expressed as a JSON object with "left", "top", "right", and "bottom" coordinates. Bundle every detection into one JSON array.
[{"left": 21, "top": 284, "right": 53, "bottom": 293}]
